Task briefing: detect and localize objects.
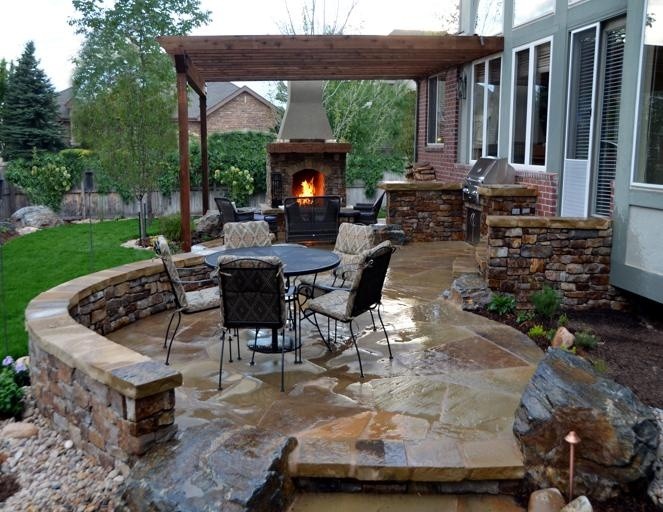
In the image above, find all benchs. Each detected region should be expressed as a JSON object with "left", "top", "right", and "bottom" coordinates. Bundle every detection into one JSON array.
[{"left": 283, "top": 195, "right": 341, "bottom": 245}]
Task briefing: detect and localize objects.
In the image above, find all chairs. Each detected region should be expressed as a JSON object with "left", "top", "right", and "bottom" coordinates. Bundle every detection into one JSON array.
[
  {"left": 216, "top": 254, "right": 298, "bottom": 391},
  {"left": 353, "top": 190, "right": 386, "bottom": 224},
  {"left": 153, "top": 234, "right": 242, "bottom": 366},
  {"left": 297, "top": 222, "right": 377, "bottom": 344},
  {"left": 296, "top": 240, "right": 394, "bottom": 378},
  {"left": 215, "top": 196, "right": 254, "bottom": 245},
  {"left": 221, "top": 221, "right": 273, "bottom": 248}
]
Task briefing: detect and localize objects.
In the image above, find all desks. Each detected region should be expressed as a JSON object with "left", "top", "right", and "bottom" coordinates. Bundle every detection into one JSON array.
[{"left": 205, "top": 245, "right": 341, "bottom": 353}]
